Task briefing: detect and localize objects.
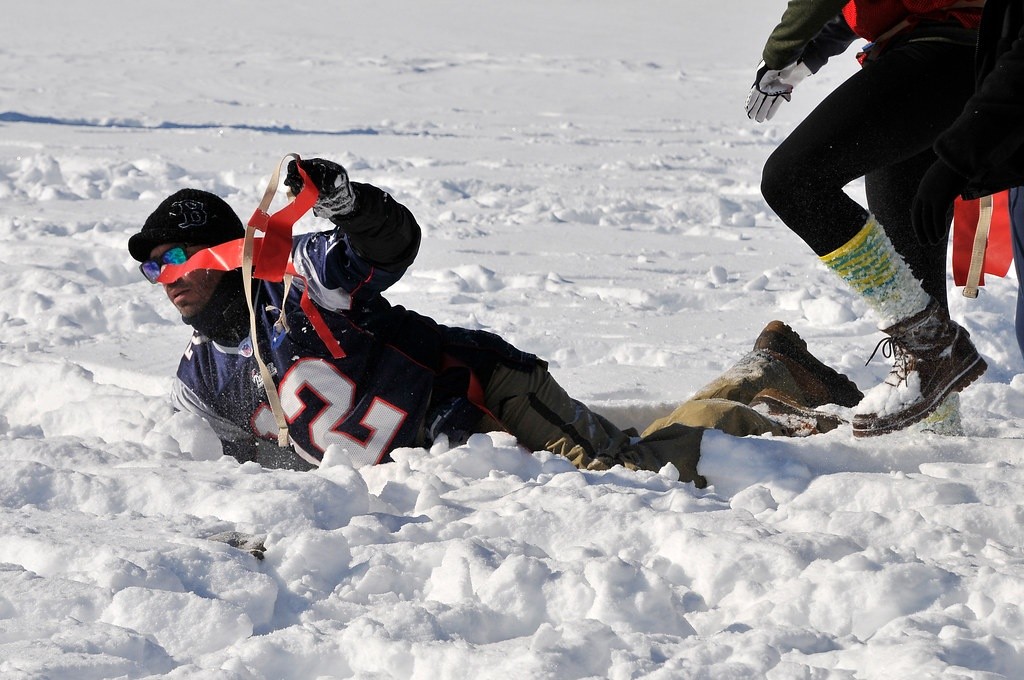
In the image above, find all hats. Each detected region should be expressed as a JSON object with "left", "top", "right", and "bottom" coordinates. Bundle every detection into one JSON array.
[{"left": 128, "top": 189, "right": 246, "bottom": 262}]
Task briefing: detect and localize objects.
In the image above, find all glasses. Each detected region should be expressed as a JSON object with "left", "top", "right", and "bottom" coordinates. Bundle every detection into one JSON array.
[{"left": 139, "top": 243, "right": 191, "bottom": 284}]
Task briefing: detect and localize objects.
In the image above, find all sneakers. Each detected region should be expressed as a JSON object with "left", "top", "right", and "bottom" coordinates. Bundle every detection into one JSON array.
[
  {"left": 853, "top": 296, "right": 988, "bottom": 437},
  {"left": 913, "top": 392, "right": 965, "bottom": 437},
  {"left": 748, "top": 388, "right": 850, "bottom": 437},
  {"left": 751, "top": 320, "right": 865, "bottom": 408}
]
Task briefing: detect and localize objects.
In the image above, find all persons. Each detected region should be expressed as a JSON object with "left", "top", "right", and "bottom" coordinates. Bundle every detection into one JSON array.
[
  {"left": 127, "top": 156, "right": 865, "bottom": 488},
  {"left": 744, "top": 1, "right": 1023, "bottom": 439}
]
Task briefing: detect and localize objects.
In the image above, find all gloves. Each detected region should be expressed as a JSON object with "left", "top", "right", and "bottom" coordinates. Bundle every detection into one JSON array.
[
  {"left": 284, "top": 158, "right": 356, "bottom": 219},
  {"left": 743, "top": 59, "right": 811, "bottom": 124},
  {"left": 911, "top": 160, "right": 965, "bottom": 247}
]
